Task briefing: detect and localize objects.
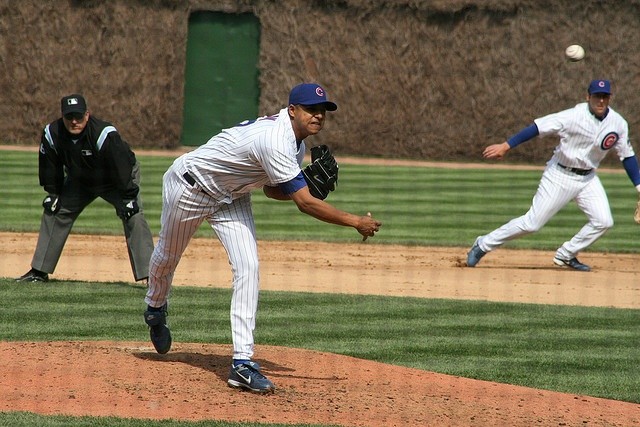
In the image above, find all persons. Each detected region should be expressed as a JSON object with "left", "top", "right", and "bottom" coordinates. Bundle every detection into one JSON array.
[
  {"left": 466, "top": 81, "right": 640, "bottom": 270},
  {"left": 13, "top": 94, "right": 155, "bottom": 286},
  {"left": 144, "top": 83, "right": 382, "bottom": 394}
]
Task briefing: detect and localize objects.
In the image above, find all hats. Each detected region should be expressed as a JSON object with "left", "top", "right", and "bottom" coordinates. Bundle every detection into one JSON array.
[
  {"left": 61, "top": 94, "right": 86, "bottom": 115},
  {"left": 588, "top": 80, "right": 611, "bottom": 93},
  {"left": 289, "top": 83, "right": 337, "bottom": 111}
]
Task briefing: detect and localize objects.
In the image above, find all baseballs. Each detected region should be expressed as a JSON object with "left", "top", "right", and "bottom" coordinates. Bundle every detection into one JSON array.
[{"left": 565, "top": 44, "right": 585, "bottom": 62}]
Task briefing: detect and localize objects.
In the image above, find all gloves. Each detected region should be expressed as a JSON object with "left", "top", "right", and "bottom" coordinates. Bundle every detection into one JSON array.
[
  {"left": 43, "top": 194, "right": 61, "bottom": 214},
  {"left": 118, "top": 198, "right": 139, "bottom": 220}
]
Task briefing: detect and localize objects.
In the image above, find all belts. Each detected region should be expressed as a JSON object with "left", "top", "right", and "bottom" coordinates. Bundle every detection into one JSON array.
[
  {"left": 558, "top": 162, "right": 592, "bottom": 175},
  {"left": 183, "top": 171, "right": 209, "bottom": 196}
]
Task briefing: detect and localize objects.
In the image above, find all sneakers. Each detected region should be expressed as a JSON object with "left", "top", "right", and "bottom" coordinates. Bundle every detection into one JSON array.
[
  {"left": 144, "top": 303, "right": 171, "bottom": 354},
  {"left": 553, "top": 256, "right": 590, "bottom": 271},
  {"left": 227, "top": 359, "right": 274, "bottom": 392},
  {"left": 15, "top": 269, "right": 48, "bottom": 283},
  {"left": 467, "top": 235, "right": 486, "bottom": 266}
]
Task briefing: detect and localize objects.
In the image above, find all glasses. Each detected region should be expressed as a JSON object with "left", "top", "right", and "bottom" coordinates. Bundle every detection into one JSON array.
[{"left": 63, "top": 113, "right": 84, "bottom": 122}]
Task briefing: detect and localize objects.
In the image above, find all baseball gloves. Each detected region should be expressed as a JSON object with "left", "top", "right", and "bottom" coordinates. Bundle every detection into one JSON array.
[{"left": 301, "top": 146, "right": 340, "bottom": 200}]
